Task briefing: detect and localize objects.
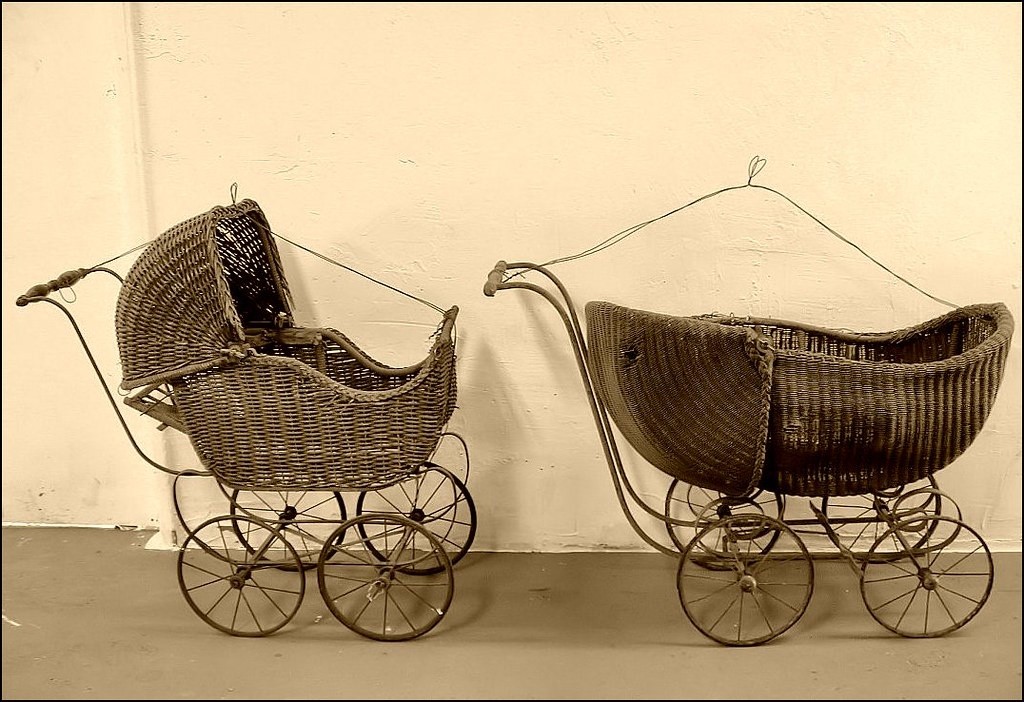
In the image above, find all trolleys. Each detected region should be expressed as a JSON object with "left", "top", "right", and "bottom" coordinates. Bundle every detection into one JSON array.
[
  {"left": 15, "top": 181, "right": 478, "bottom": 641},
  {"left": 483, "top": 154, "right": 1017, "bottom": 650}
]
